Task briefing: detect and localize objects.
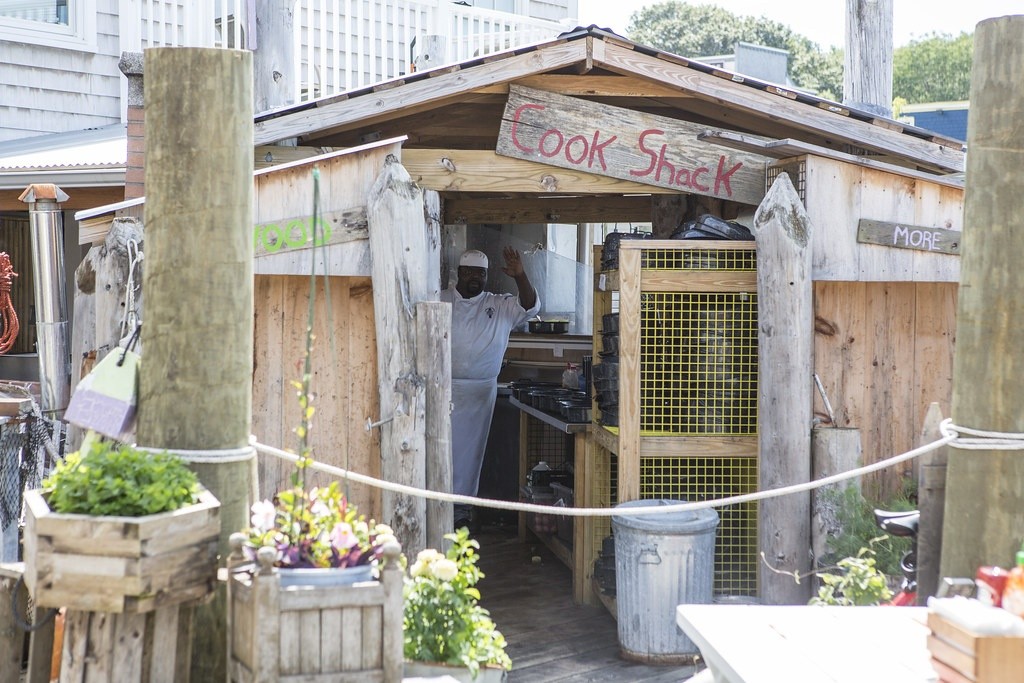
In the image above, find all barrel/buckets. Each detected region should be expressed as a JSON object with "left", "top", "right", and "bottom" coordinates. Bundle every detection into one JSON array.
[{"left": 611, "top": 498, "right": 721, "bottom": 666}]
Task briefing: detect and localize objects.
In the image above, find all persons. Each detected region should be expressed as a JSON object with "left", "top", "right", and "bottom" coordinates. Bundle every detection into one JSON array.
[{"left": 438, "top": 245, "right": 542, "bottom": 537}]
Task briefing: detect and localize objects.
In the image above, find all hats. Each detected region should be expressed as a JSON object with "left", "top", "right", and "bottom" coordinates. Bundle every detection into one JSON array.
[{"left": 459, "top": 250, "right": 488, "bottom": 268}]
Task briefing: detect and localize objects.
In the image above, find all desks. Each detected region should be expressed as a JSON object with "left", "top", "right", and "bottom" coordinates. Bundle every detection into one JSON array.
[
  {"left": 675, "top": 603, "right": 944, "bottom": 683},
  {"left": 510, "top": 397, "right": 594, "bottom": 604}
]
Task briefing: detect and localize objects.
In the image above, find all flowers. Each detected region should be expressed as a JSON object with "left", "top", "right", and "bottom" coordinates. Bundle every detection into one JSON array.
[{"left": 228, "top": 164, "right": 403, "bottom": 569}]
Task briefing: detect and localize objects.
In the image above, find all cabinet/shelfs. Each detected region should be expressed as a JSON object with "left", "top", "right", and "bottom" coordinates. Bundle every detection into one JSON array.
[{"left": 585, "top": 238, "right": 758, "bottom": 619}]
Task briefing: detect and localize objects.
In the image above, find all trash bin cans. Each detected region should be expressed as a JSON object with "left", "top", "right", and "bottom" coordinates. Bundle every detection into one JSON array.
[{"left": 608, "top": 497, "right": 719, "bottom": 666}]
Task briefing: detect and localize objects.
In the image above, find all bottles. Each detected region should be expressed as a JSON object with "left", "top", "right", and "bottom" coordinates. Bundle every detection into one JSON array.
[
  {"left": 562, "top": 361, "right": 578, "bottom": 389},
  {"left": 972, "top": 549, "right": 1024, "bottom": 621}
]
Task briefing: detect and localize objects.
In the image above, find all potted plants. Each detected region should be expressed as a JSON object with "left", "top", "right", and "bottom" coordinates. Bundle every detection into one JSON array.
[{"left": 21, "top": 432, "right": 224, "bottom": 615}]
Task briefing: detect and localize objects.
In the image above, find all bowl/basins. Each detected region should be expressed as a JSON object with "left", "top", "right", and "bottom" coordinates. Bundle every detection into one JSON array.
[{"left": 509, "top": 380, "right": 593, "bottom": 423}]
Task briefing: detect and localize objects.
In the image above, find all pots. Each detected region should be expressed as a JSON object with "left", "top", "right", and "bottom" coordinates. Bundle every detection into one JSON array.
[
  {"left": 524, "top": 320, "right": 571, "bottom": 334},
  {"left": 593, "top": 535, "right": 618, "bottom": 597},
  {"left": 600, "top": 231, "right": 654, "bottom": 268},
  {"left": 593, "top": 313, "right": 619, "bottom": 427}
]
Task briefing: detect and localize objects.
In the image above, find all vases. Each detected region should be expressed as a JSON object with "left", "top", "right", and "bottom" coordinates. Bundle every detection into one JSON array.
[{"left": 252, "top": 559, "right": 378, "bottom": 586}]
